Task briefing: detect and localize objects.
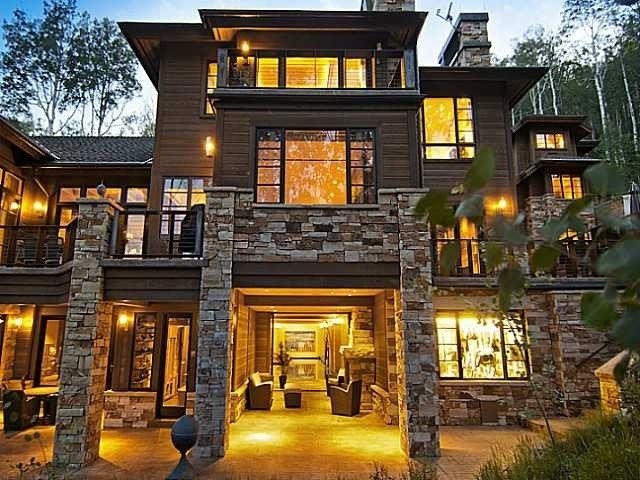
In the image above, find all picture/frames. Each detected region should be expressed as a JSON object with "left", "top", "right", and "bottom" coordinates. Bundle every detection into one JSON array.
[
  {"left": 284, "top": 389, "right": 302, "bottom": 409},
  {"left": 24, "top": 386, "right": 60, "bottom": 422}
]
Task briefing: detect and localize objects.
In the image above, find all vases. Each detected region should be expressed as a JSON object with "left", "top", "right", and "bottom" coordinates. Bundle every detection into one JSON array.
[{"left": 271, "top": 338, "right": 293, "bottom": 375}]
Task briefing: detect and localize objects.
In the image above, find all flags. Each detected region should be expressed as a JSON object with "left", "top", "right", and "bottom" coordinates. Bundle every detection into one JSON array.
[{"left": 279, "top": 374, "right": 287, "bottom": 389}]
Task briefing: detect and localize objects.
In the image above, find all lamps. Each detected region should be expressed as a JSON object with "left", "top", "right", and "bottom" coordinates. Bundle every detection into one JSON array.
[
  {"left": 329, "top": 378, "right": 363, "bottom": 417},
  {"left": 2, "top": 389, "right": 41, "bottom": 431}
]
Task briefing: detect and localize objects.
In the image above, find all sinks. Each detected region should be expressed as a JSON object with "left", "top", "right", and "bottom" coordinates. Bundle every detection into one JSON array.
[{"left": 249, "top": 370, "right": 274, "bottom": 411}]
